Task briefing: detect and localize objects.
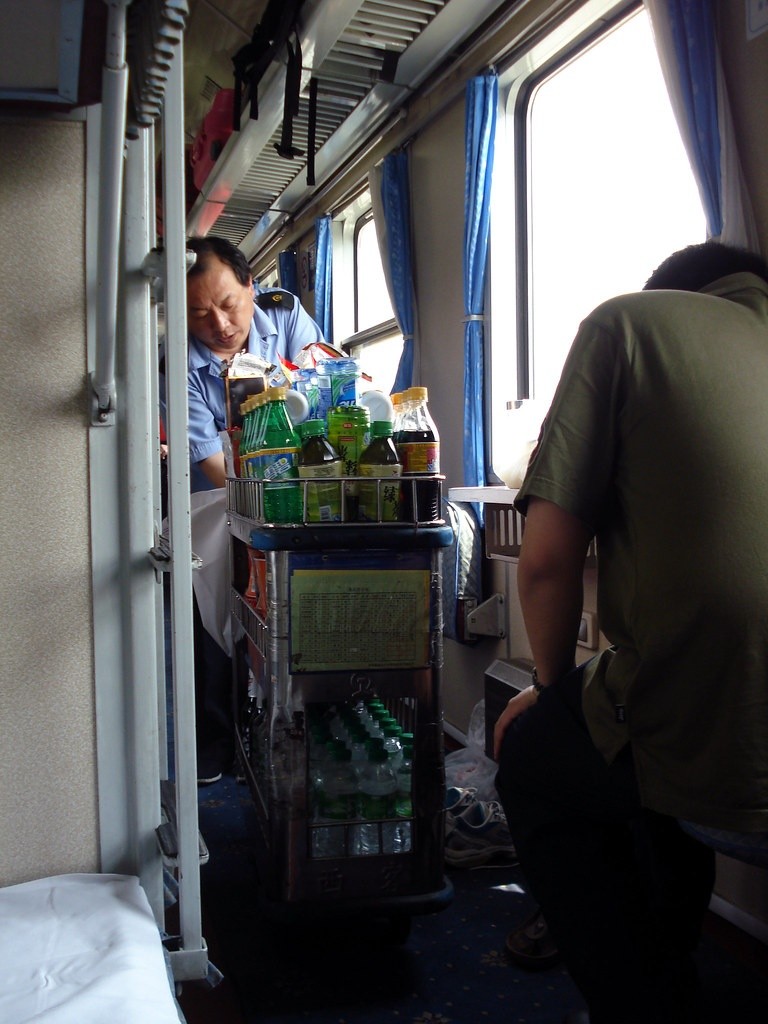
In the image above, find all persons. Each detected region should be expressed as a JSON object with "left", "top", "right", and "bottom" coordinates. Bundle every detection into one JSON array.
[
  {"left": 159, "top": 236, "right": 428, "bottom": 775},
  {"left": 493, "top": 239, "right": 768, "bottom": 1024}
]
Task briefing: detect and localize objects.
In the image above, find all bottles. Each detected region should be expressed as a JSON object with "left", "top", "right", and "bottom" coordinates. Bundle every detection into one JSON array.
[
  {"left": 236, "top": 359, "right": 440, "bottom": 528},
  {"left": 237, "top": 688, "right": 416, "bottom": 858}
]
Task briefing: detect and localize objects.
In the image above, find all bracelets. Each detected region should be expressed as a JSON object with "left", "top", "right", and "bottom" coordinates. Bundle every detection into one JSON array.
[{"left": 532, "top": 667, "right": 544, "bottom": 691}]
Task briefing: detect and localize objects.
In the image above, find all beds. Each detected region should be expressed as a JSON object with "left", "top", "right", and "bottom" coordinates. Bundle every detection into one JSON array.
[{"left": 0, "top": 873, "right": 185, "bottom": 1024}]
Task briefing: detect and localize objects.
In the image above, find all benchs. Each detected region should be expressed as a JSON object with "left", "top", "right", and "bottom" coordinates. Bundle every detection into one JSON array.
[{"left": 678, "top": 821, "right": 768, "bottom": 993}]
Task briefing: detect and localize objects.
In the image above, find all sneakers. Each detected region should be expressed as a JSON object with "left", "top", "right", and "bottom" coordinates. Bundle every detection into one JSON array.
[{"left": 443, "top": 787, "right": 519, "bottom": 868}]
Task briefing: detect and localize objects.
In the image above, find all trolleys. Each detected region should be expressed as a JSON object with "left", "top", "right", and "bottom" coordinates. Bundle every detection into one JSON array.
[{"left": 223, "top": 473, "right": 459, "bottom": 954}]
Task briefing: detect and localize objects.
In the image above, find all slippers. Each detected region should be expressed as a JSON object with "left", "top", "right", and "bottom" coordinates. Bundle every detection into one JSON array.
[{"left": 504, "top": 904, "right": 556, "bottom": 969}]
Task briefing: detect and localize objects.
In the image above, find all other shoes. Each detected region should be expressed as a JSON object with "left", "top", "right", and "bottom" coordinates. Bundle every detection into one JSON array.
[{"left": 198, "top": 748, "right": 222, "bottom": 784}]
